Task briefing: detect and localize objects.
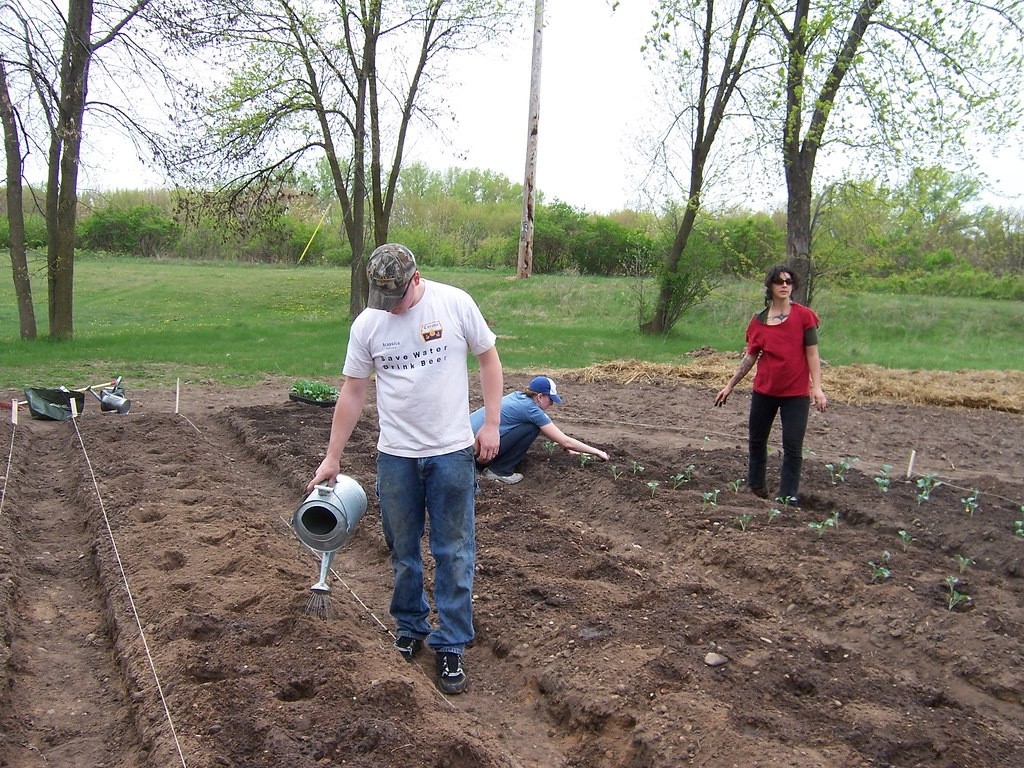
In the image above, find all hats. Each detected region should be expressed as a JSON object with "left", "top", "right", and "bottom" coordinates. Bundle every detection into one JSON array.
[
  {"left": 528, "top": 377, "right": 562, "bottom": 403},
  {"left": 366, "top": 243, "right": 417, "bottom": 311}
]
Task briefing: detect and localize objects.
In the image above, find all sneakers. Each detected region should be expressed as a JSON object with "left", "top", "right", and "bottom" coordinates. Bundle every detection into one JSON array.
[
  {"left": 393, "top": 636, "right": 424, "bottom": 659},
  {"left": 436, "top": 652, "right": 467, "bottom": 694},
  {"left": 481, "top": 468, "right": 523, "bottom": 484}
]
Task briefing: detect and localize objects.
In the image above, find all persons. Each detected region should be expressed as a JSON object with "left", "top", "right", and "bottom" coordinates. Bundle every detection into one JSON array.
[
  {"left": 714, "top": 267, "right": 826, "bottom": 503},
  {"left": 469, "top": 377, "right": 609, "bottom": 485},
  {"left": 307, "top": 243, "right": 503, "bottom": 695}
]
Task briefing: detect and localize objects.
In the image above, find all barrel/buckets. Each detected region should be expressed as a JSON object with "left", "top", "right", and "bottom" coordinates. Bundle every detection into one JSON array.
[{"left": 102, "top": 376, "right": 124, "bottom": 411}]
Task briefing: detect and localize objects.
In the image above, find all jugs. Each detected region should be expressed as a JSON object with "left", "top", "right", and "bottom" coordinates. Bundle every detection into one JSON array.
[
  {"left": 85, "top": 385, "right": 131, "bottom": 415},
  {"left": 294, "top": 474, "right": 367, "bottom": 595}
]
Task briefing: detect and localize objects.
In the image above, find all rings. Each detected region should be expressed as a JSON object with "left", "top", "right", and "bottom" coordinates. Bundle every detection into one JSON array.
[{"left": 493, "top": 452, "right": 496, "bottom": 454}]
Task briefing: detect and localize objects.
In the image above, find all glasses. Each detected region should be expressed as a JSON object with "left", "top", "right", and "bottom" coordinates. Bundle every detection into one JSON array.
[{"left": 773, "top": 278, "right": 794, "bottom": 285}]
